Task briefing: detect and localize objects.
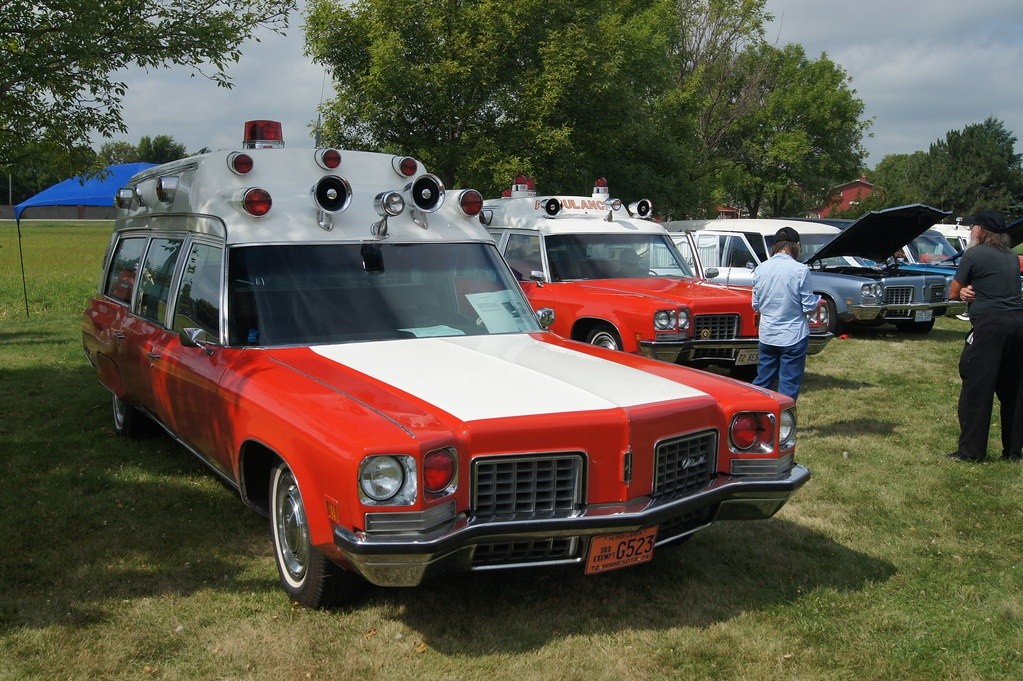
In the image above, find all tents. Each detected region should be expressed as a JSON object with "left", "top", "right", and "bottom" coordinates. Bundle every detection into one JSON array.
[{"left": 14, "top": 161, "right": 175, "bottom": 318}]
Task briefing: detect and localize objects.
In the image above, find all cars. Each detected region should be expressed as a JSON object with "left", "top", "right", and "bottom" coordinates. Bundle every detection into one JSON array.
[
  {"left": 436, "top": 175, "right": 760, "bottom": 382},
  {"left": 585, "top": 201, "right": 969, "bottom": 337},
  {"left": 1017, "top": 255, "right": 1023, "bottom": 290},
  {"left": 771, "top": 217, "right": 965, "bottom": 275},
  {"left": 80, "top": 118, "right": 810, "bottom": 609},
  {"left": 919, "top": 223, "right": 972, "bottom": 255}
]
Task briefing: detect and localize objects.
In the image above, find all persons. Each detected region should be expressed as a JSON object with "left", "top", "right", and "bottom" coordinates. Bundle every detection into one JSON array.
[
  {"left": 750, "top": 226, "right": 821, "bottom": 401},
  {"left": 617, "top": 248, "right": 649, "bottom": 274},
  {"left": 946, "top": 208, "right": 1023, "bottom": 463}
]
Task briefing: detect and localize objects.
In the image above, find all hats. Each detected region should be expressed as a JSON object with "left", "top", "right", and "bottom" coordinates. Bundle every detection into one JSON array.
[
  {"left": 961, "top": 208, "right": 1006, "bottom": 234},
  {"left": 773, "top": 226, "right": 803, "bottom": 254}
]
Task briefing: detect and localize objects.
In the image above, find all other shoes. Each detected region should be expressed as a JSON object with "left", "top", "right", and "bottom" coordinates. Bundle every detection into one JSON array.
[
  {"left": 947, "top": 451, "right": 978, "bottom": 462},
  {"left": 997, "top": 455, "right": 1016, "bottom": 461}
]
